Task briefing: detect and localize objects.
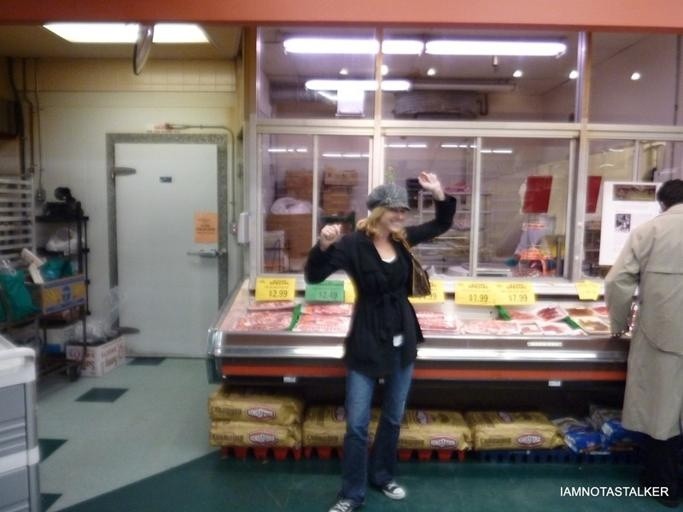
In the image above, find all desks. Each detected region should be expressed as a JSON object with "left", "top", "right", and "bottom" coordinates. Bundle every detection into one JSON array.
[{"left": 0, "top": 314, "right": 40, "bottom": 334}]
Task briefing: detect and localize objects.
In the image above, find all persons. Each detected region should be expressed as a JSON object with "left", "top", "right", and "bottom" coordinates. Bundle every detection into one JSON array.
[
  {"left": 604, "top": 180, "right": 683, "bottom": 507},
  {"left": 305, "top": 171, "right": 456, "bottom": 512}
]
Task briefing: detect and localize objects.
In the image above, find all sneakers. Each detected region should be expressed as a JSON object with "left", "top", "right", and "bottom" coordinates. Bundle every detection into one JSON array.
[
  {"left": 328, "top": 496, "right": 361, "bottom": 512},
  {"left": 379, "top": 479, "right": 406, "bottom": 501}
]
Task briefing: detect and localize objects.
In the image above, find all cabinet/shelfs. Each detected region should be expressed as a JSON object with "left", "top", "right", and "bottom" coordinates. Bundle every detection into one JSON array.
[
  {"left": 33, "top": 214, "right": 91, "bottom": 383},
  {"left": 418, "top": 184, "right": 494, "bottom": 265}
]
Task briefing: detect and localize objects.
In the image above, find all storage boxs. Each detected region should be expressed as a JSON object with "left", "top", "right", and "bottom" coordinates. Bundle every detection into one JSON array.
[
  {"left": 23, "top": 272, "right": 88, "bottom": 316},
  {"left": 266, "top": 168, "right": 359, "bottom": 259},
  {"left": 65, "top": 335, "right": 126, "bottom": 376}
]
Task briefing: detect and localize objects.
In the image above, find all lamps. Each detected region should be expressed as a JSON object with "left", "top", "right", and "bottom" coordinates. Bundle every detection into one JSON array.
[{"left": 281, "top": 34, "right": 569, "bottom": 91}]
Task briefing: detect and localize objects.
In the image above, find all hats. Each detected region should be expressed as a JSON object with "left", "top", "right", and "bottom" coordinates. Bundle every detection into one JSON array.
[{"left": 365, "top": 182, "right": 410, "bottom": 211}]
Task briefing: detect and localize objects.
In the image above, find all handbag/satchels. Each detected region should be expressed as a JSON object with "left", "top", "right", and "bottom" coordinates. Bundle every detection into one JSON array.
[{"left": 411, "top": 255, "right": 431, "bottom": 297}]
